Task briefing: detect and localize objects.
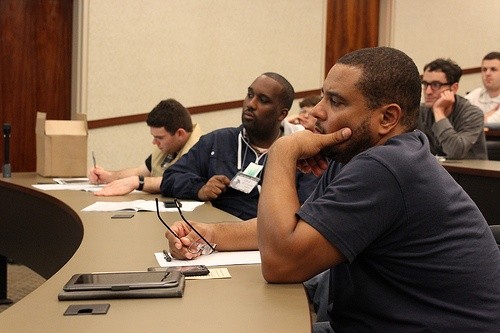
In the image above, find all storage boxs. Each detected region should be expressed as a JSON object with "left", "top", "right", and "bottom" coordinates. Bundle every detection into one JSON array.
[{"left": 35, "top": 112, "right": 88, "bottom": 178}]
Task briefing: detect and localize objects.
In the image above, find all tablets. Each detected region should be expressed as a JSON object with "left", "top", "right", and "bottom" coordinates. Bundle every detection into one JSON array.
[{"left": 63, "top": 272, "right": 181, "bottom": 287}]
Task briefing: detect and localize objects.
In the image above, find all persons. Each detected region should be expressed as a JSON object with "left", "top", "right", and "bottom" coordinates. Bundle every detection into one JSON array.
[
  {"left": 417, "top": 58, "right": 488, "bottom": 159},
  {"left": 286, "top": 96, "right": 322, "bottom": 132},
  {"left": 159, "top": 71, "right": 324, "bottom": 221},
  {"left": 89, "top": 98, "right": 202, "bottom": 197},
  {"left": 166, "top": 47, "right": 500, "bottom": 332},
  {"left": 462, "top": 53, "right": 500, "bottom": 129}
]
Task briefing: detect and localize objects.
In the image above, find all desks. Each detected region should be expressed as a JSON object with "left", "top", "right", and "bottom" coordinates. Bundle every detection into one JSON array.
[
  {"left": 437, "top": 128, "right": 500, "bottom": 225},
  {"left": 0, "top": 170, "right": 312, "bottom": 333}
]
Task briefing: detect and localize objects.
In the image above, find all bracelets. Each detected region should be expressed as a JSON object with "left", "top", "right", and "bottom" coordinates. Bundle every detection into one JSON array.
[{"left": 136, "top": 175, "right": 144, "bottom": 190}]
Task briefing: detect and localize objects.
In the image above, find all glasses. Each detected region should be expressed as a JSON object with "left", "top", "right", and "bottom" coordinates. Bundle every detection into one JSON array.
[
  {"left": 421, "top": 81, "right": 449, "bottom": 90},
  {"left": 156, "top": 198, "right": 217, "bottom": 255}
]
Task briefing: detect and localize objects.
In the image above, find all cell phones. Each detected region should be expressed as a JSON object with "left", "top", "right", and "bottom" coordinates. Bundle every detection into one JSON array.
[{"left": 148, "top": 265, "right": 209, "bottom": 276}]
